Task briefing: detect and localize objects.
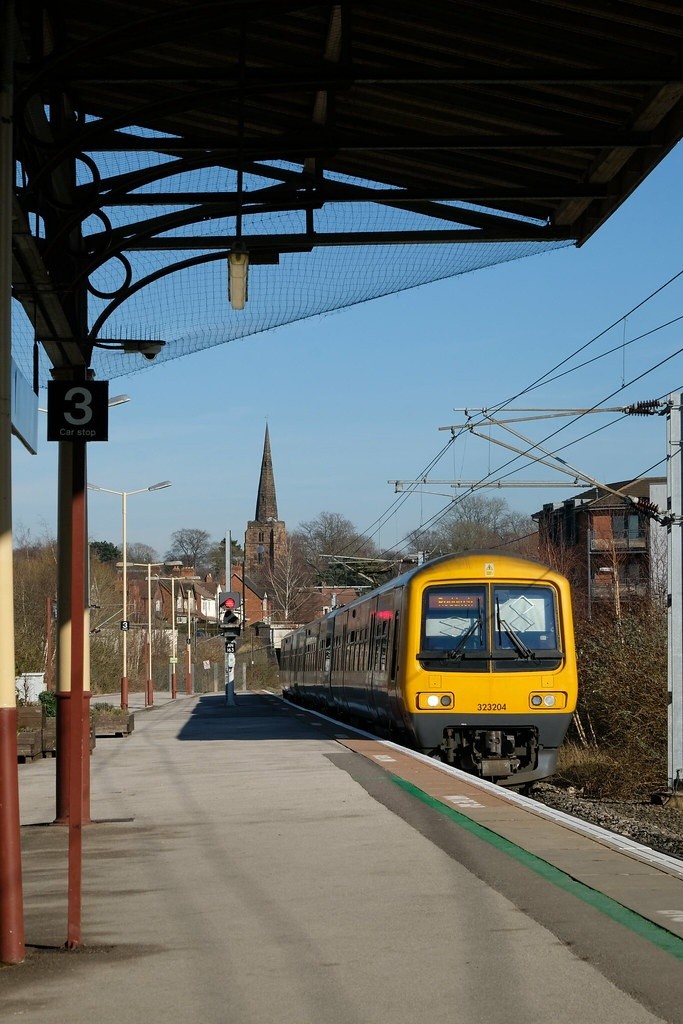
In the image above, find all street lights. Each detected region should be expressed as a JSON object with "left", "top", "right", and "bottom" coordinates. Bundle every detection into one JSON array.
[
  {"left": 88, "top": 481, "right": 173, "bottom": 711},
  {"left": 143, "top": 575, "right": 202, "bottom": 700},
  {"left": 116, "top": 560, "right": 183, "bottom": 707},
  {"left": 186, "top": 589, "right": 193, "bottom": 695}
]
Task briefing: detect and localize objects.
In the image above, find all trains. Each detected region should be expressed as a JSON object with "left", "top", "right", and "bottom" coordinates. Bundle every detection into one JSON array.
[{"left": 278, "top": 551, "right": 579, "bottom": 788}]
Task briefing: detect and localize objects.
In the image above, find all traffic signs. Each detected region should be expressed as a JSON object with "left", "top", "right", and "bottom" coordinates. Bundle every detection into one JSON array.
[{"left": 218, "top": 592, "right": 242, "bottom": 640}]
